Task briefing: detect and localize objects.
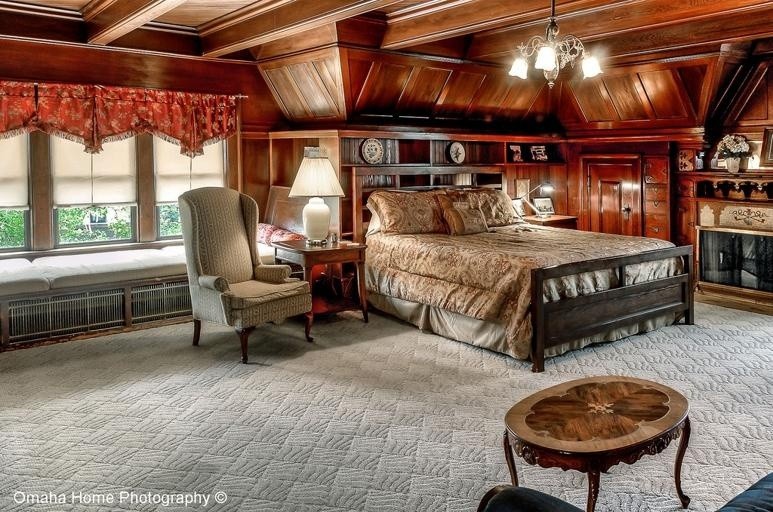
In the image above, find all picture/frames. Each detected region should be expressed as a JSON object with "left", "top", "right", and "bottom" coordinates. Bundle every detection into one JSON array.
[
  {"left": 516, "top": 178, "right": 530, "bottom": 202},
  {"left": 534, "top": 197, "right": 555, "bottom": 214},
  {"left": 512, "top": 198, "right": 527, "bottom": 216},
  {"left": 759, "top": 126, "right": 773, "bottom": 166}
]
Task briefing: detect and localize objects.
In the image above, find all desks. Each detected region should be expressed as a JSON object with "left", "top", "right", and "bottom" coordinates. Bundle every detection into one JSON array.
[{"left": 502, "top": 375, "right": 691, "bottom": 512}]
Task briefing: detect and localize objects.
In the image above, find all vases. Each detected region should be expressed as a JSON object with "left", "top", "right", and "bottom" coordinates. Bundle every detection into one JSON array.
[{"left": 726, "top": 157, "right": 740, "bottom": 173}]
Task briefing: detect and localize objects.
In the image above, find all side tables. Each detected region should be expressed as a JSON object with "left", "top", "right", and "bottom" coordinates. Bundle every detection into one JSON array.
[{"left": 271, "top": 241, "right": 368, "bottom": 324}]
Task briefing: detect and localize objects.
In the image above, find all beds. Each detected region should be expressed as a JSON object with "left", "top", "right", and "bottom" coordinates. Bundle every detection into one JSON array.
[
  {"left": 0, "top": 186, "right": 303, "bottom": 348},
  {"left": 352, "top": 166, "right": 695, "bottom": 373}
]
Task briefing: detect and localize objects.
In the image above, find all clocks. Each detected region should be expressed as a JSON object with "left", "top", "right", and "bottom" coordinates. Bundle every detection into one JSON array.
[{"left": 449, "top": 142, "right": 466, "bottom": 163}]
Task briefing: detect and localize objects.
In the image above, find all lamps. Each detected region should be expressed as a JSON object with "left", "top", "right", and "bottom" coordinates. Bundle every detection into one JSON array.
[
  {"left": 289, "top": 158, "right": 346, "bottom": 245},
  {"left": 508, "top": 0, "right": 603, "bottom": 89},
  {"left": 522, "top": 182, "right": 556, "bottom": 218}
]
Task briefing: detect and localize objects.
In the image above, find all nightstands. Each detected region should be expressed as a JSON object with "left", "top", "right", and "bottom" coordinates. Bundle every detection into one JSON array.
[{"left": 524, "top": 214, "right": 577, "bottom": 229}]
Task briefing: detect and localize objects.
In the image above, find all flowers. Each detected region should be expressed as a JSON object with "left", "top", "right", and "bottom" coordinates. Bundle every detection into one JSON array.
[{"left": 718, "top": 135, "right": 749, "bottom": 157}]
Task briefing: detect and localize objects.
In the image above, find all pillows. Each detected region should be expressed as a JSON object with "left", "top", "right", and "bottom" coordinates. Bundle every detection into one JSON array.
[
  {"left": 367, "top": 186, "right": 522, "bottom": 235},
  {"left": 256, "top": 223, "right": 304, "bottom": 249}
]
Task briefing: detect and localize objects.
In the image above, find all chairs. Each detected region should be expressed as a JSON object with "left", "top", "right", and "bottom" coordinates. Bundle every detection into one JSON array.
[{"left": 179, "top": 188, "right": 314, "bottom": 363}]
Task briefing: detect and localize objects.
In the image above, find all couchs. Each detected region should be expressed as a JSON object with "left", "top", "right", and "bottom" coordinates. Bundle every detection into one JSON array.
[{"left": 477, "top": 463, "right": 772, "bottom": 512}]
